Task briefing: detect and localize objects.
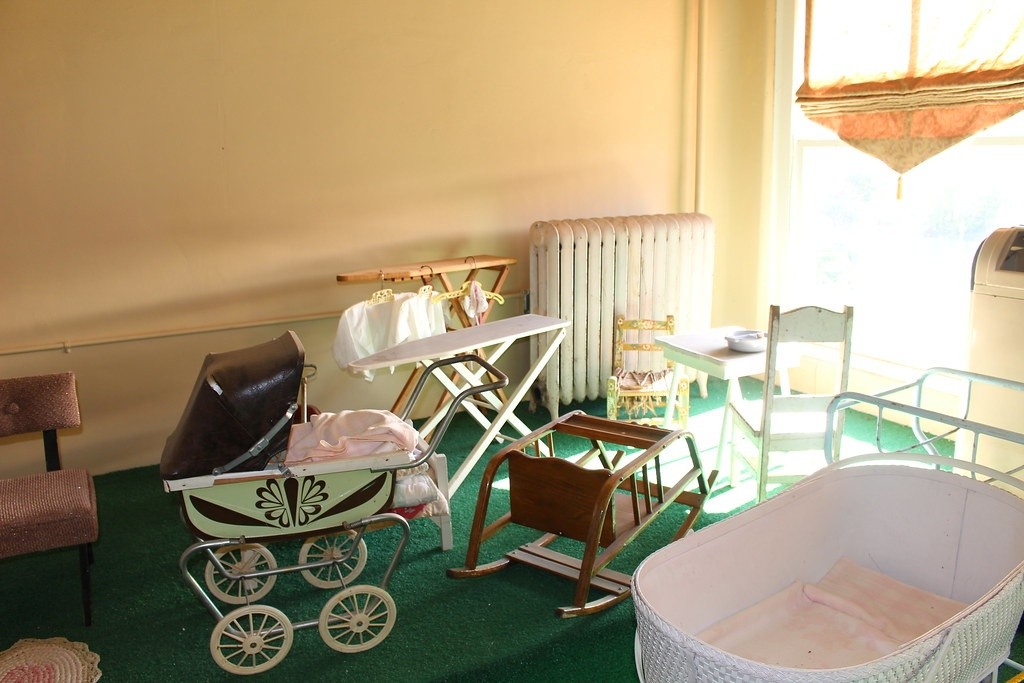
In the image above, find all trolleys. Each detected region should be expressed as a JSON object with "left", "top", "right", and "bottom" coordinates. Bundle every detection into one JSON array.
[{"left": 156, "top": 351, "right": 510, "bottom": 676}]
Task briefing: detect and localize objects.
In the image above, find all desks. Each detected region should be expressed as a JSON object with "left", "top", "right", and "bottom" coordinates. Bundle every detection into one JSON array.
[
  {"left": 348, "top": 313, "right": 571, "bottom": 507},
  {"left": 336, "top": 254, "right": 520, "bottom": 451},
  {"left": 654, "top": 328, "right": 799, "bottom": 467}
]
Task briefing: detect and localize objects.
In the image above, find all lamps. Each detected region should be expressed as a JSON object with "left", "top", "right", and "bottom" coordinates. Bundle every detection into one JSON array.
[{"left": 797, "top": 0, "right": 1024, "bottom": 198}]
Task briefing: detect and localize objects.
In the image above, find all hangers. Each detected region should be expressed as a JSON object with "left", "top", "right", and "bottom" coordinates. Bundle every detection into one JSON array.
[{"left": 365, "top": 257, "right": 508, "bottom": 315}]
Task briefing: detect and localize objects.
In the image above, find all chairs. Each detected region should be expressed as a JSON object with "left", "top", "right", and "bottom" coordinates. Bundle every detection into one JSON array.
[
  {"left": 725, "top": 304, "right": 853, "bottom": 504},
  {"left": 0, "top": 372, "right": 98, "bottom": 628},
  {"left": 606, "top": 315, "right": 690, "bottom": 429}
]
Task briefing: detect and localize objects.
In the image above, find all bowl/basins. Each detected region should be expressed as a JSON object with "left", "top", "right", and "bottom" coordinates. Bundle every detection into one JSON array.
[{"left": 725, "top": 330, "right": 768, "bottom": 353}]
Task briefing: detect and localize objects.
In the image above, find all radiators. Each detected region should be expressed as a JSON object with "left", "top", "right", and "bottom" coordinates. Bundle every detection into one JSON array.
[{"left": 530, "top": 213, "right": 714, "bottom": 418}]
